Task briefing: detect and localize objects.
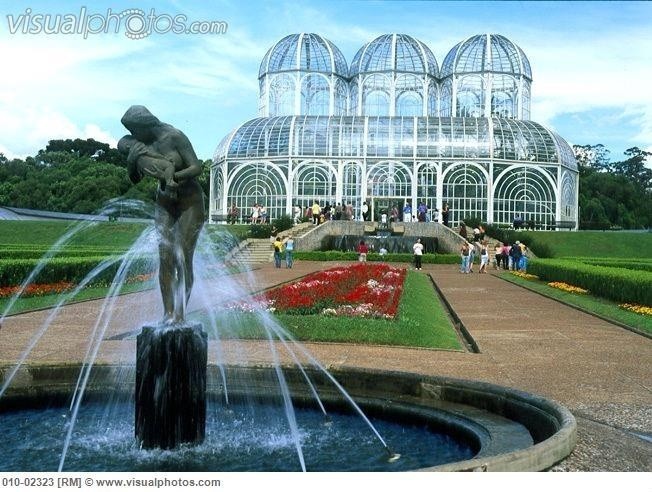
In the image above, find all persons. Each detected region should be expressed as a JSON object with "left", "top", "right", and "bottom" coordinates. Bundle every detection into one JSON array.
[
  {"left": 359, "top": 240, "right": 368, "bottom": 262},
  {"left": 294, "top": 201, "right": 439, "bottom": 226},
  {"left": 273, "top": 237, "right": 286, "bottom": 268},
  {"left": 284, "top": 235, "right": 295, "bottom": 267},
  {"left": 413, "top": 238, "right": 424, "bottom": 269},
  {"left": 121, "top": 106, "right": 206, "bottom": 324},
  {"left": 459, "top": 215, "right": 527, "bottom": 274},
  {"left": 260, "top": 205, "right": 267, "bottom": 223},
  {"left": 252, "top": 204, "right": 259, "bottom": 224},
  {"left": 270, "top": 224, "right": 277, "bottom": 248},
  {"left": 227, "top": 203, "right": 238, "bottom": 223},
  {"left": 118, "top": 136, "right": 178, "bottom": 201}
]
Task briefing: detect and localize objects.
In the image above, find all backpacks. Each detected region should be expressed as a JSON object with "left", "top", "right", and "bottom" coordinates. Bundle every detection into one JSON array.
[
  {"left": 481, "top": 245, "right": 487, "bottom": 254},
  {"left": 275, "top": 245, "right": 280, "bottom": 252}
]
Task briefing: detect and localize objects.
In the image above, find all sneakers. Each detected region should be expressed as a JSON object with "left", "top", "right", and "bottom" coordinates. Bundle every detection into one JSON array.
[
  {"left": 495, "top": 266, "right": 526, "bottom": 272},
  {"left": 415, "top": 267, "right": 423, "bottom": 271},
  {"left": 459, "top": 267, "right": 487, "bottom": 274},
  {"left": 275, "top": 264, "right": 293, "bottom": 269}
]
